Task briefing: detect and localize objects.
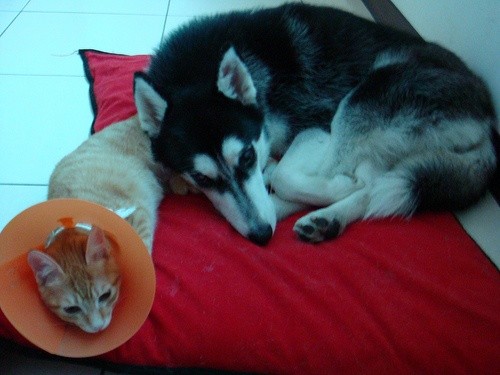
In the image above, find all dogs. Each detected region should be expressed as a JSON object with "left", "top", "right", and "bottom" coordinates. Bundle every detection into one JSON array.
[{"left": 131, "top": 2, "right": 499, "bottom": 247}]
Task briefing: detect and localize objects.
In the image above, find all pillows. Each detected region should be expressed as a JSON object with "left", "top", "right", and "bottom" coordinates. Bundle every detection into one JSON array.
[{"left": 0, "top": 48, "right": 500, "bottom": 375}]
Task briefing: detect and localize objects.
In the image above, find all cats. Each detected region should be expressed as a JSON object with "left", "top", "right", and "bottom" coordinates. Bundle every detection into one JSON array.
[{"left": 27, "top": 113, "right": 200, "bottom": 333}]
